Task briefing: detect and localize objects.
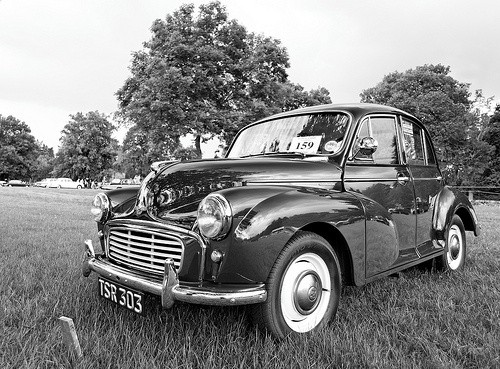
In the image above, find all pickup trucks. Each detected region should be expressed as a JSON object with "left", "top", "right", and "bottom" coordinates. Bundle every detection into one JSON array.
[{"left": 102, "top": 178, "right": 141, "bottom": 190}]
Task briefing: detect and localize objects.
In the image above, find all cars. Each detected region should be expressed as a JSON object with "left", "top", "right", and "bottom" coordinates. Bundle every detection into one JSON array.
[
  {"left": 81, "top": 102, "right": 480, "bottom": 344},
  {"left": 34, "top": 177, "right": 84, "bottom": 190},
  {"left": 4, "top": 180, "right": 32, "bottom": 188}
]
